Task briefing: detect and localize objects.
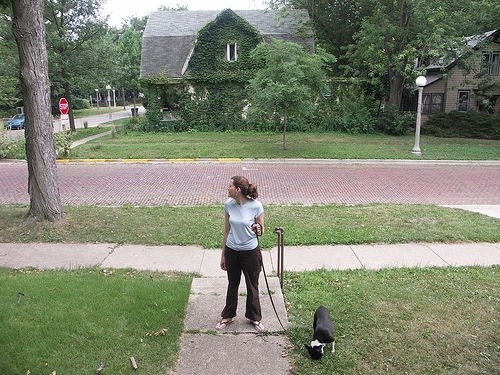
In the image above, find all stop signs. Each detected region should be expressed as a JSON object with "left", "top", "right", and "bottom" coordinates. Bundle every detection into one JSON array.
[{"left": 59, "top": 98, "right": 69, "bottom": 114}]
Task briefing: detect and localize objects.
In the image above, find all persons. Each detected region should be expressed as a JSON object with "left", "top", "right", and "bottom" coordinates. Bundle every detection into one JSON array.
[{"left": 215, "top": 175, "right": 265, "bottom": 334}]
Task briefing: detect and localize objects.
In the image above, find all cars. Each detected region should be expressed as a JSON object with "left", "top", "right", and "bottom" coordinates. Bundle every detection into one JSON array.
[{"left": 4, "top": 114, "right": 25, "bottom": 130}]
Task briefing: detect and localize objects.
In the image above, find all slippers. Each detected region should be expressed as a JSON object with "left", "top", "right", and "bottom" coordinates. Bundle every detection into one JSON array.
[
  {"left": 250, "top": 320, "right": 264, "bottom": 332},
  {"left": 216, "top": 318, "right": 234, "bottom": 330}
]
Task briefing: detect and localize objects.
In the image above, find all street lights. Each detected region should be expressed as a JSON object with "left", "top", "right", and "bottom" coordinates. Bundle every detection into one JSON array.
[{"left": 411, "top": 76, "right": 427, "bottom": 154}]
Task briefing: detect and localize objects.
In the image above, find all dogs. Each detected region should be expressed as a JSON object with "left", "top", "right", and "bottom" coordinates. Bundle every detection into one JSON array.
[{"left": 303, "top": 305, "right": 335, "bottom": 360}]
